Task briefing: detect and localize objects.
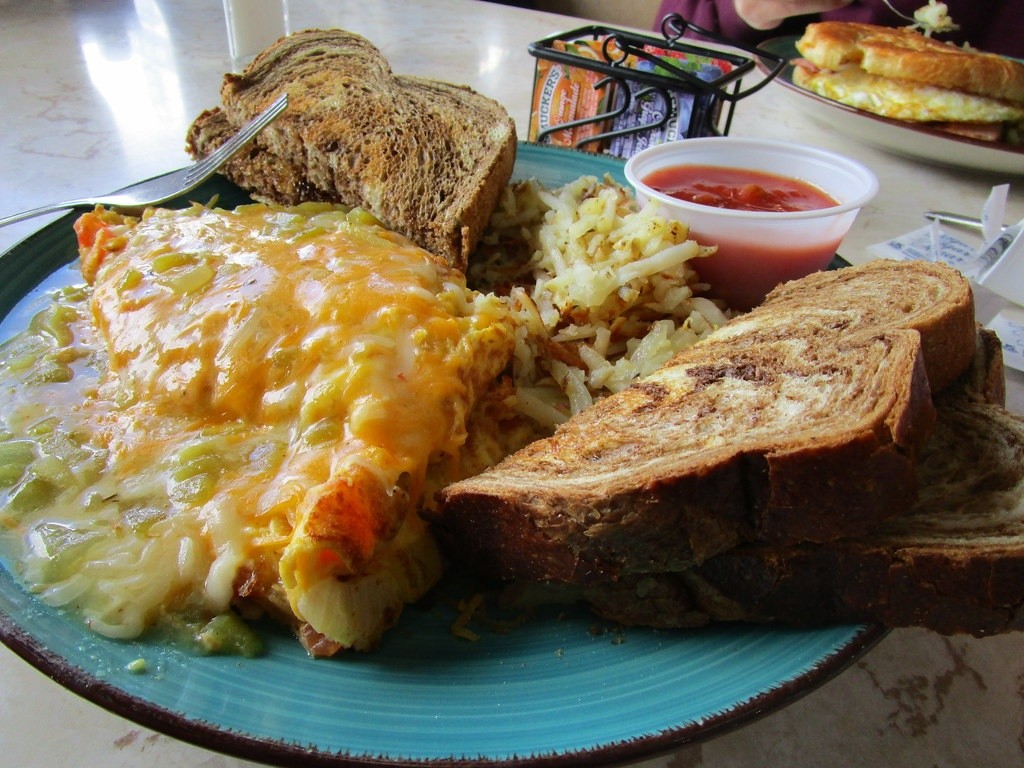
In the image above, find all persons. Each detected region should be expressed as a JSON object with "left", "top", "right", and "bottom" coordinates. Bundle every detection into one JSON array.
[{"left": 652, "top": 0, "right": 1024, "bottom": 66}]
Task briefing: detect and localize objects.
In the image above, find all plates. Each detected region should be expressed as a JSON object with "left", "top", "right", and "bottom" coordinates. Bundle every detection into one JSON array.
[
  {"left": 754, "top": 37, "right": 1024, "bottom": 177},
  {"left": 0, "top": 138, "right": 898, "bottom": 768}
]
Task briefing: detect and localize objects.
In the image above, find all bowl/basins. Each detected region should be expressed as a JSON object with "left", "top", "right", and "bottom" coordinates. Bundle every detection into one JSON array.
[{"left": 624, "top": 136, "right": 881, "bottom": 316}]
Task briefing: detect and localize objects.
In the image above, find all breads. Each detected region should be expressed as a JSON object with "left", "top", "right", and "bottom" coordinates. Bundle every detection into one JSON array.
[
  {"left": 441, "top": 259, "right": 978, "bottom": 584},
  {"left": 184, "top": 108, "right": 331, "bottom": 212},
  {"left": 222, "top": 30, "right": 518, "bottom": 261},
  {"left": 508, "top": 317, "right": 1024, "bottom": 633},
  {"left": 795, "top": 22, "right": 1024, "bottom": 102}
]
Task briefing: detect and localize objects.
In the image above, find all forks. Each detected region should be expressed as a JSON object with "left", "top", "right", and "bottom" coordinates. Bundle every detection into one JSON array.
[{"left": 0, "top": 91, "right": 289, "bottom": 228}]
